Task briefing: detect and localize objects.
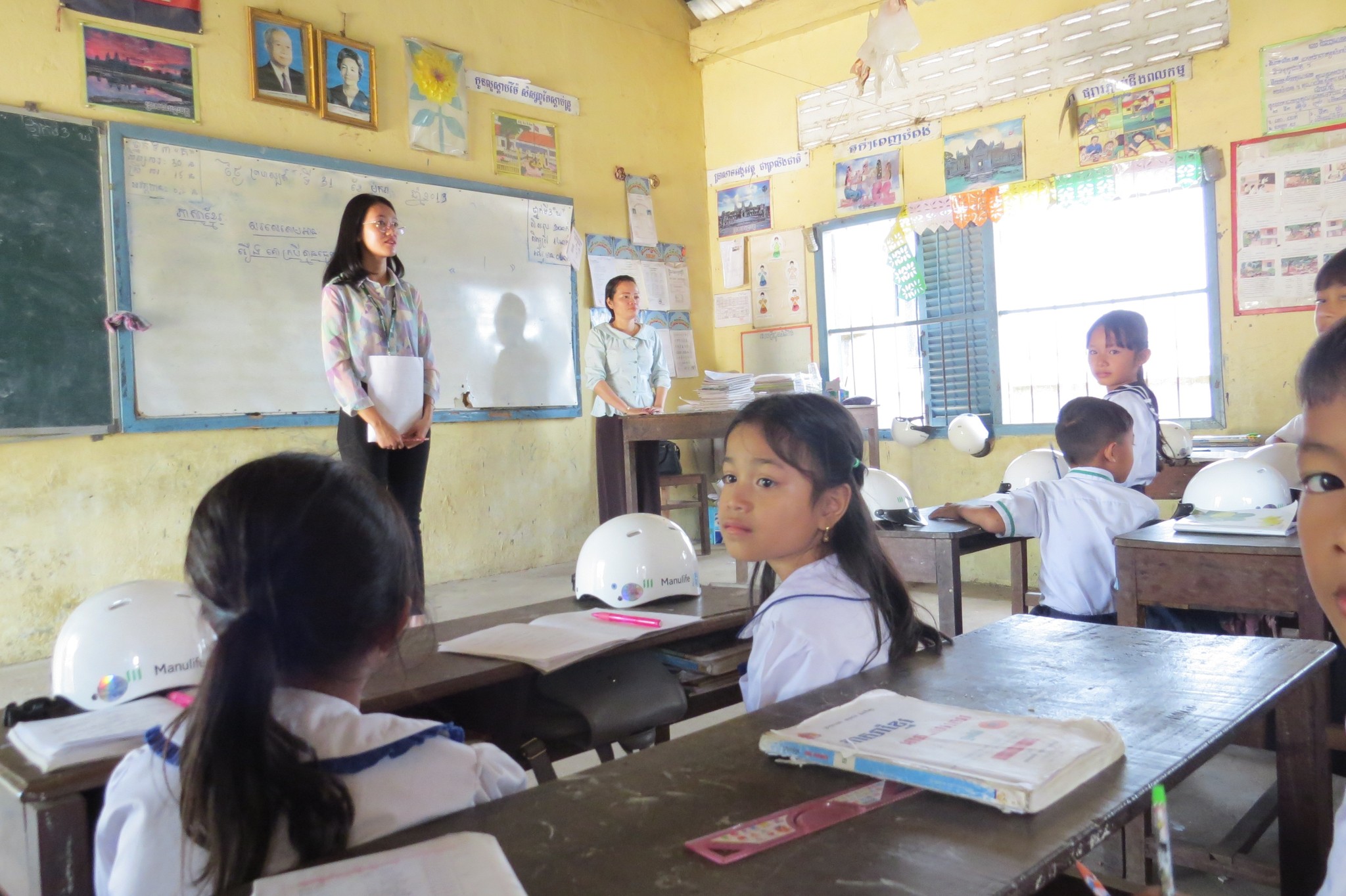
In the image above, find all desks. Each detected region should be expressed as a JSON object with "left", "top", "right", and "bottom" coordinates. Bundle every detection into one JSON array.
[
  {"left": 213, "top": 613, "right": 1338, "bottom": 896},
  {"left": 857, "top": 496, "right": 1047, "bottom": 641},
  {"left": 617, "top": 402, "right": 882, "bottom": 516},
  {"left": 1140, "top": 457, "right": 1210, "bottom": 499},
  {"left": 1112, "top": 506, "right": 1325, "bottom": 643},
  {"left": 0, "top": 582, "right": 774, "bottom": 896}
]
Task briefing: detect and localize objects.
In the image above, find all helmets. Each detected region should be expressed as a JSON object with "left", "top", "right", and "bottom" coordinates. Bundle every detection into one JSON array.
[
  {"left": 1157, "top": 421, "right": 1192, "bottom": 462},
  {"left": 51, "top": 578, "right": 222, "bottom": 709},
  {"left": 1001, "top": 448, "right": 1071, "bottom": 496},
  {"left": 1181, "top": 458, "right": 1292, "bottom": 513},
  {"left": 892, "top": 418, "right": 934, "bottom": 448},
  {"left": 948, "top": 413, "right": 991, "bottom": 458},
  {"left": 574, "top": 512, "right": 700, "bottom": 612},
  {"left": 859, "top": 468, "right": 927, "bottom": 529},
  {"left": 1244, "top": 442, "right": 1303, "bottom": 489}
]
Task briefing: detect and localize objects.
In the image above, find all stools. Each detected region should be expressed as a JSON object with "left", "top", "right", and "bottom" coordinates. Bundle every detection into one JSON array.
[{"left": 657, "top": 472, "right": 712, "bottom": 556}]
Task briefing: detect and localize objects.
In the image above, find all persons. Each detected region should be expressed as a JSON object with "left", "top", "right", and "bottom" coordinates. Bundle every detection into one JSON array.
[
  {"left": 1290, "top": 309, "right": 1346, "bottom": 896},
  {"left": 584, "top": 275, "right": 671, "bottom": 525},
  {"left": 322, "top": 192, "right": 444, "bottom": 627},
  {"left": 90, "top": 451, "right": 529, "bottom": 896},
  {"left": 1264, "top": 248, "right": 1346, "bottom": 441},
  {"left": 1087, "top": 307, "right": 1176, "bottom": 497},
  {"left": 718, "top": 394, "right": 955, "bottom": 713},
  {"left": 326, "top": 47, "right": 370, "bottom": 112},
  {"left": 928, "top": 396, "right": 1161, "bottom": 623},
  {"left": 255, "top": 24, "right": 306, "bottom": 97}
]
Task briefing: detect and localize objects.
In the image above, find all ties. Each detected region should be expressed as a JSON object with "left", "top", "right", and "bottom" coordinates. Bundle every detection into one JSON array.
[{"left": 281, "top": 72, "right": 290, "bottom": 92}]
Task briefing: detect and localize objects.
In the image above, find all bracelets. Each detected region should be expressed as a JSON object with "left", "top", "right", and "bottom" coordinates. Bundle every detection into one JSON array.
[{"left": 624, "top": 406, "right": 631, "bottom": 414}]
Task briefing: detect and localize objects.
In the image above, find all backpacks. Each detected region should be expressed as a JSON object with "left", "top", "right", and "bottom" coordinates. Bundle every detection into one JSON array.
[{"left": 503, "top": 649, "right": 692, "bottom": 780}]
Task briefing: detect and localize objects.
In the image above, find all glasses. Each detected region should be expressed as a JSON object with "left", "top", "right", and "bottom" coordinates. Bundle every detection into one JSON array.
[{"left": 363, "top": 219, "right": 406, "bottom": 234}]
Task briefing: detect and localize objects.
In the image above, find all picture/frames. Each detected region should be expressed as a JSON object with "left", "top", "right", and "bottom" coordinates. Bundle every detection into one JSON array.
[
  {"left": 247, "top": 7, "right": 317, "bottom": 113},
  {"left": 317, "top": 30, "right": 379, "bottom": 131}
]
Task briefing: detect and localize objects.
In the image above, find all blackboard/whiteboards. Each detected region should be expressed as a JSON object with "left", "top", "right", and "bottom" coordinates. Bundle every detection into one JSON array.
[
  {"left": 105, "top": 119, "right": 581, "bottom": 432},
  {"left": 0, "top": 98, "right": 119, "bottom": 438}
]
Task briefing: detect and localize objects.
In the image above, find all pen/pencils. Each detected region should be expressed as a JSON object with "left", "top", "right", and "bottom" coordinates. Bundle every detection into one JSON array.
[
  {"left": 165, "top": 690, "right": 194, "bottom": 709},
  {"left": 1076, "top": 859, "right": 1112, "bottom": 896},
  {"left": 1150, "top": 784, "right": 1175, "bottom": 896},
  {"left": 591, "top": 612, "right": 662, "bottom": 628}
]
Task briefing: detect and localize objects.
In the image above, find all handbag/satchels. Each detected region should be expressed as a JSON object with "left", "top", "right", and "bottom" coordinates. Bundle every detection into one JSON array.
[{"left": 656, "top": 440, "right": 683, "bottom": 477}]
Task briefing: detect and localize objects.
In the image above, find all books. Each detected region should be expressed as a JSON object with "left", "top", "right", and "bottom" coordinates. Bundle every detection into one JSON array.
[
  {"left": 1173, "top": 499, "right": 1300, "bottom": 536},
  {"left": 437, "top": 607, "right": 701, "bottom": 671},
  {"left": 1191, "top": 434, "right": 1266, "bottom": 463},
  {"left": 250, "top": 828, "right": 531, "bottom": 896},
  {"left": 7, "top": 687, "right": 201, "bottom": 775},
  {"left": 751, "top": 373, "right": 820, "bottom": 393},
  {"left": 760, "top": 686, "right": 1128, "bottom": 817}
]
